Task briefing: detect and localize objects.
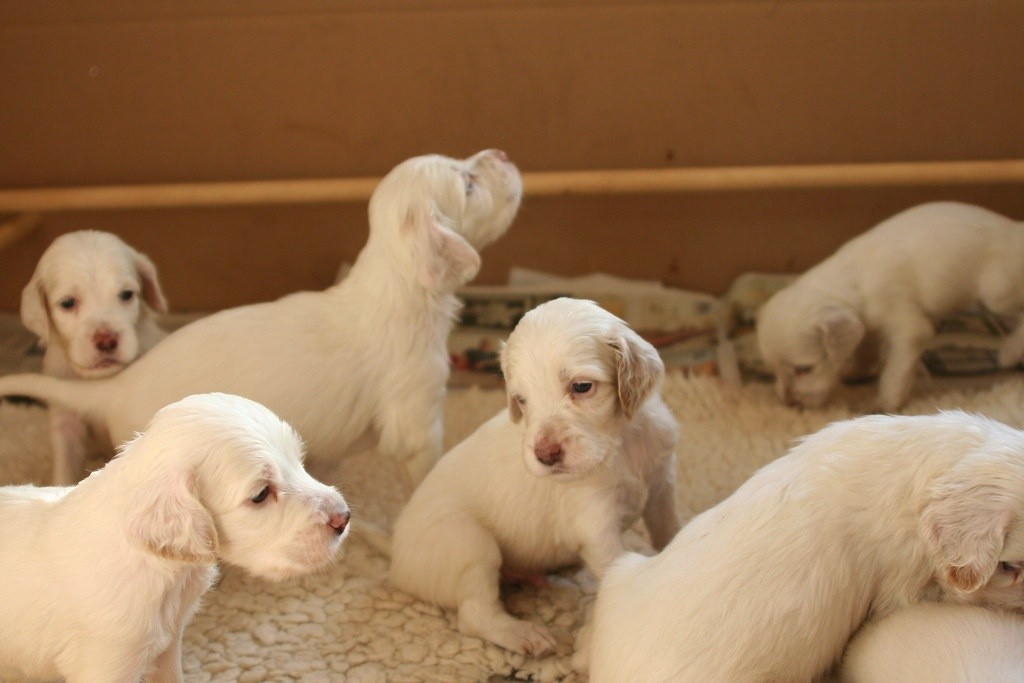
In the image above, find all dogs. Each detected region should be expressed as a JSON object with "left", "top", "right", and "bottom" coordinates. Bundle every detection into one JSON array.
[
  {"left": 755, "top": 199, "right": 1022, "bottom": 415},
  {"left": 350, "top": 297, "right": 681, "bottom": 660},
  {"left": 21, "top": 230, "right": 168, "bottom": 485},
  {"left": 0, "top": 390, "right": 351, "bottom": 682},
  {"left": 572, "top": 406, "right": 1023, "bottom": 683},
  {"left": 0, "top": 149, "right": 524, "bottom": 494}
]
